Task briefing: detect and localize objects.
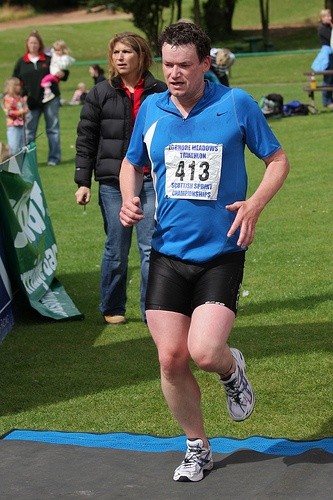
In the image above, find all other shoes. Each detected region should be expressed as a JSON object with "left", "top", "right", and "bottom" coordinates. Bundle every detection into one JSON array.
[{"left": 103, "top": 314, "right": 126, "bottom": 324}]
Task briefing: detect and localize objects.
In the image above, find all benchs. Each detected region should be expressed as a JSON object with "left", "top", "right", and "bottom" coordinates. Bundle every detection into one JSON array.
[{"left": 302, "top": 70, "right": 333, "bottom": 107}]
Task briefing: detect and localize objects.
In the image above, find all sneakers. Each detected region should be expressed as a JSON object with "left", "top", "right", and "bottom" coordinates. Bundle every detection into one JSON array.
[
  {"left": 173, "top": 438, "right": 214, "bottom": 483},
  {"left": 219, "top": 347, "right": 256, "bottom": 422}
]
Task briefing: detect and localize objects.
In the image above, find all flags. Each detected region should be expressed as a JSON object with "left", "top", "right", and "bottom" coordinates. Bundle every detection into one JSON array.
[{"left": 0, "top": 142, "right": 83, "bottom": 321}]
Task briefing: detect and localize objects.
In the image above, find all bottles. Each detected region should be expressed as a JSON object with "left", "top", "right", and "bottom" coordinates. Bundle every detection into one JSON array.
[{"left": 310, "top": 72, "right": 316, "bottom": 89}]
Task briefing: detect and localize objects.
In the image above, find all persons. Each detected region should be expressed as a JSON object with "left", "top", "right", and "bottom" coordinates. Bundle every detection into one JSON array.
[
  {"left": 74, "top": 31, "right": 173, "bottom": 326},
  {"left": 40, "top": 39, "right": 76, "bottom": 103},
  {"left": 81, "top": 62, "right": 107, "bottom": 101},
  {"left": 9, "top": 30, "right": 70, "bottom": 166},
  {"left": 1, "top": 77, "right": 31, "bottom": 158},
  {"left": 208, "top": 47, "right": 236, "bottom": 88},
  {"left": 318, "top": 8, "right": 333, "bottom": 109},
  {"left": 118, "top": 21, "right": 290, "bottom": 484},
  {"left": 72, "top": 83, "right": 86, "bottom": 101}
]
